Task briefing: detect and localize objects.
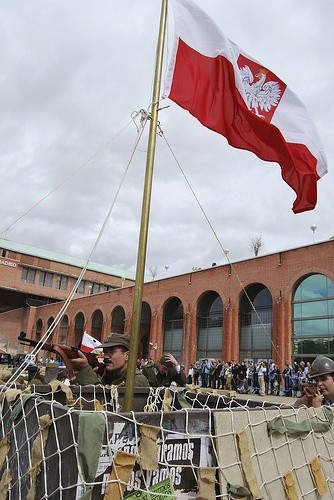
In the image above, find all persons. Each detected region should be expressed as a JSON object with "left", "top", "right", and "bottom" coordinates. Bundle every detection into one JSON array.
[
  {"left": 67, "top": 333, "right": 150, "bottom": 387},
  {"left": 142, "top": 352, "right": 186, "bottom": 387},
  {"left": 134, "top": 356, "right": 188, "bottom": 375},
  {"left": 292, "top": 355, "right": 334, "bottom": 406},
  {"left": 1, "top": 353, "right": 65, "bottom": 373},
  {"left": 187, "top": 358, "right": 311, "bottom": 397}
]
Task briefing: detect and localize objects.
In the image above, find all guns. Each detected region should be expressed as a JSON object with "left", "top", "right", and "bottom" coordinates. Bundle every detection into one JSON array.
[
  {"left": 290, "top": 382, "right": 322, "bottom": 403},
  {"left": 17, "top": 331, "right": 104, "bottom": 376}
]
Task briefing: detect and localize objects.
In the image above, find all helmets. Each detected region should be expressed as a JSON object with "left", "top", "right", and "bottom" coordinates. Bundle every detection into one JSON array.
[{"left": 308, "top": 355, "right": 334, "bottom": 379}]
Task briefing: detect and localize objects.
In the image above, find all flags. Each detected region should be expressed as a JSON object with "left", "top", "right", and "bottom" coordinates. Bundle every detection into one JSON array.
[
  {"left": 162, "top": 0, "right": 328, "bottom": 216},
  {"left": 80, "top": 332, "right": 110, "bottom": 366}
]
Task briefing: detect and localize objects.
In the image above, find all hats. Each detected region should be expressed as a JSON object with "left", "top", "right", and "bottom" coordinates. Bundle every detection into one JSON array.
[
  {"left": 95, "top": 332, "right": 130, "bottom": 351},
  {"left": 12, "top": 367, "right": 21, "bottom": 375},
  {"left": 28, "top": 366, "right": 40, "bottom": 372},
  {"left": 160, "top": 352, "right": 174, "bottom": 368}
]
